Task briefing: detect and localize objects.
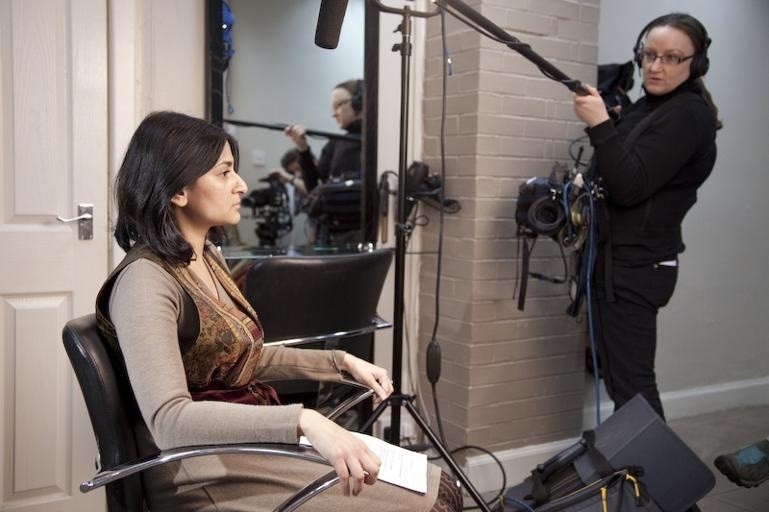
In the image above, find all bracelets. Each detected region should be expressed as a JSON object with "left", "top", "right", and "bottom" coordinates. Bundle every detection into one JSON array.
[{"left": 288, "top": 177, "right": 296, "bottom": 185}]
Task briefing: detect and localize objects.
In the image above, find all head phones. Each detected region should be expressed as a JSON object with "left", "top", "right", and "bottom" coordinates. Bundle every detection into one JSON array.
[
  {"left": 351, "top": 79, "right": 362, "bottom": 113},
  {"left": 633, "top": 14, "right": 712, "bottom": 81}
]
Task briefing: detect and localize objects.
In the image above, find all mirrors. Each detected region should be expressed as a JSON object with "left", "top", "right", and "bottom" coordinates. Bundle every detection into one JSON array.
[{"left": 204, "top": 1, "right": 379, "bottom": 254}]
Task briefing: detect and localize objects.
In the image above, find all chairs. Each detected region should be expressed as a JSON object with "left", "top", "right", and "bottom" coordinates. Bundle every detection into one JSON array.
[
  {"left": 62, "top": 313, "right": 375, "bottom": 511},
  {"left": 245, "top": 246, "right": 394, "bottom": 440}
]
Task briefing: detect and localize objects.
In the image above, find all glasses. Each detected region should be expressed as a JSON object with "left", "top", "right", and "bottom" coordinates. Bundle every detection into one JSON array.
[
  {"left": 640, "top": 51, "right": 693, "bottom": 65},
  {"left": 334, "top": 98, "right": 352, "bottom": 110}
]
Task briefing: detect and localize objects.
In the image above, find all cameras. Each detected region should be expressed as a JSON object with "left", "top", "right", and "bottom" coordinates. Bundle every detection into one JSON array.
[{"left": 242, "top": 173, "right": 290, "bottom": 218}]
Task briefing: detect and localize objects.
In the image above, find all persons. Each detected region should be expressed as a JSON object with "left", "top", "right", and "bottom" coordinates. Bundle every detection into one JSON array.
[
  {"left": 573, "top": 12, "right": 721, "bottom": 427},
  {"left": 100, "top": 113, "right": 462, "bottom": 512},
  {"left": 286, "top": 79, "right": 364, "bottom": 198},
  {"left": 274, "top": 148, "right": 316, "bottom": 251}
]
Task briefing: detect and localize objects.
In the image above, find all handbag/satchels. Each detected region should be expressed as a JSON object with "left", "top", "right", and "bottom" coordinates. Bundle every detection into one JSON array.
[{"left": 503, "top": 395, "right": 715, "bottom": 511}]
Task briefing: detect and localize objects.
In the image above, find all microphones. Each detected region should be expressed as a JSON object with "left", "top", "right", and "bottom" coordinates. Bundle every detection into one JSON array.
[{"left": 315, "top": 0, "right": 348, "bottom": 49}]
